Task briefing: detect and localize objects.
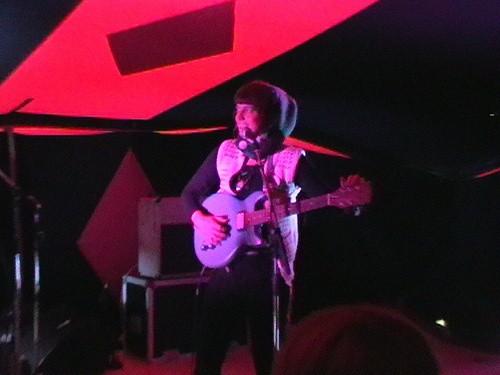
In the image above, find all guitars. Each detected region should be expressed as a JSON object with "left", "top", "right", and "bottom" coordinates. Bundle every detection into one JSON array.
[{"left": 194, "top": 175, "right": 372, "bottom": 269}]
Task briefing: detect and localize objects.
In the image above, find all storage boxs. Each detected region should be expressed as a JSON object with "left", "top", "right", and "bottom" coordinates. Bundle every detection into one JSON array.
[
  {"left": 117, "top": 273, "right": 255, "bottom": 362},
  {"left": 134, "top": 196, "right": 212, "bottom": 279}
]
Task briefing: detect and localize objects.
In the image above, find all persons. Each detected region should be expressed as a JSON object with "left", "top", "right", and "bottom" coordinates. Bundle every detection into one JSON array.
[
  {"left": 181, "top": 79, "right": 361, "bottom": 375},
  {"left": 273, "top": 303, "right": 443, "bottom": 375}
]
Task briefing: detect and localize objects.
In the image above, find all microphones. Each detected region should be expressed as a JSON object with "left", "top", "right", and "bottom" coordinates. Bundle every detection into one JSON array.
[{"left": 241, "top": 127, "right": 259, "bottom": 148}]
